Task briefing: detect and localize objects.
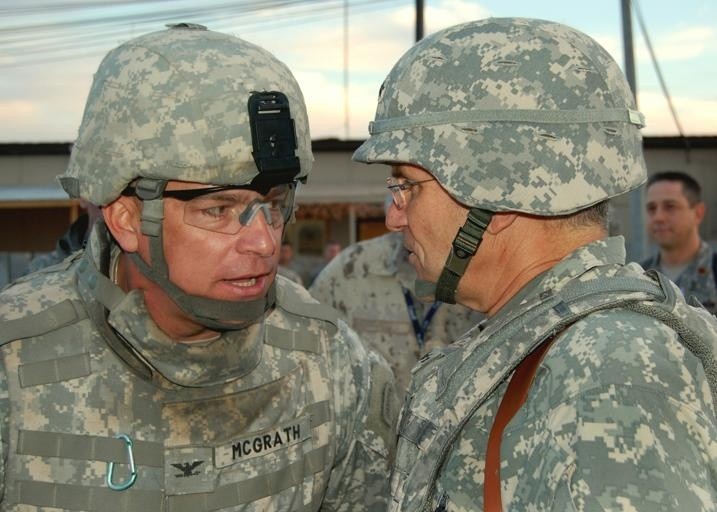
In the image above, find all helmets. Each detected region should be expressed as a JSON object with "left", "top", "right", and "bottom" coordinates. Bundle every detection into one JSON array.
[
  {"left": 56, "top": 25, "right": 314, "bottom": 209},
  {"left": 351, "top": 17, "right": 649, "bottom": 219}
]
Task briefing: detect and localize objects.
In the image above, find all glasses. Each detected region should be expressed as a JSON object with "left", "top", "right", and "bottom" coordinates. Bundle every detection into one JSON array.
[
  {"left": 383, "top": 174, "right": 436, "bottom": 211},
  {"left": 120, "top": 179, "right": 298, "bottom": 237}
]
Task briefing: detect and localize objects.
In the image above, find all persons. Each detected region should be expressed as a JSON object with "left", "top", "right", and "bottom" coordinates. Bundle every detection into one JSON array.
[
  {"left": 278, "top": 240, "right": 311, "bottom": 290},
  {"left": 312, "top": 230, "right": 488, "bottom": 408},
  {"left": 19, "top": 192, "right": 102, "bottom": 277},
  {"left": 306, "top": 239, "right": 342, "bottom": 288},
  {"left": 0, "top": 22, "right": 400, "bottom": 511},
  {"left": 352, "top": 16, "right": 716, "bottom": 512},
  {"left": 637, "top": 172, "right": 717, "bottom": 320}
]
